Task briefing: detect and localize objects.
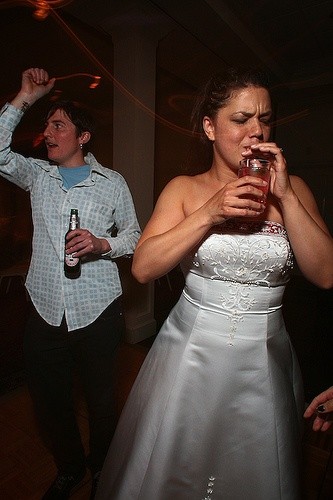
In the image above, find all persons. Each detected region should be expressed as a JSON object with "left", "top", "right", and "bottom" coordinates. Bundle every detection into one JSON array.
[
  {"left": 0, "top": 68, "right": 144, "bottom": 500},
  {"left": 95, "top": 66, "right": 333, "bottom": 500},
  {"left": 304, "top": 387, "right": 332, "bottom": 431}
]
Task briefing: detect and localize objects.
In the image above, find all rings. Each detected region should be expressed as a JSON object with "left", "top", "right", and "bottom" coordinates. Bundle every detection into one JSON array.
[{"left": 278, "top": 147, "right": 284, "bottom": 154}]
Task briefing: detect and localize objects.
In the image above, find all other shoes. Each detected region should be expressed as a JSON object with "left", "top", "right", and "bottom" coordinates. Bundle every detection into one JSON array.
[{"left": 42, "top": 469, "right": 84, "bottom": 500}]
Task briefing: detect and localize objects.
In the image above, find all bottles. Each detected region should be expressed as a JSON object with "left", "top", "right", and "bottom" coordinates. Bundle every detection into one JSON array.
[{"left": 64, "top": 208, "right": 81, "bottom": 279}]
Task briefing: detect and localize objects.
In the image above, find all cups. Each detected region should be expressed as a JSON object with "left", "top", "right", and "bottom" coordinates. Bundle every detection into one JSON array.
[{"left": 237, "top": 158, "right": 271, "bottom": 222}]
